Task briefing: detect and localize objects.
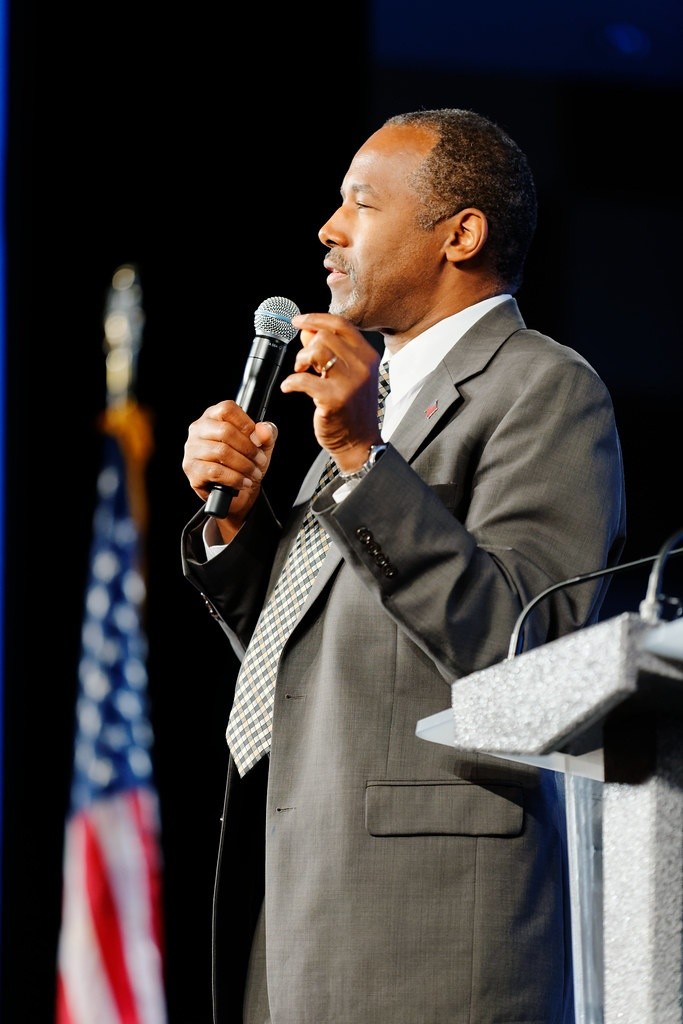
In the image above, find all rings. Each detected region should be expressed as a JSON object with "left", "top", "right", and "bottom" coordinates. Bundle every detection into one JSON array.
[{"left": 321, "top": 354, "right": 337, "bottom": 378}]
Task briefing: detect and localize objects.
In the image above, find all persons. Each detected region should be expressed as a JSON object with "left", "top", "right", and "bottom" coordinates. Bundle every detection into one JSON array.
[{"left": 181, "top": 110, "right": 628, "bottom": 1024}]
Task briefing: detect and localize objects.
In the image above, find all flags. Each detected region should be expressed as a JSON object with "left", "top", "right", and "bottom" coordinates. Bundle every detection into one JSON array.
[{"left": 56, "top": 402, "right": 173, "bottom": 1023}]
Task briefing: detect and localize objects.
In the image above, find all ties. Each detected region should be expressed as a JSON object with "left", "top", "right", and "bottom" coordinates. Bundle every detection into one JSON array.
[{"left": 224, "top": 360, "right": 392, "bottom": 783}]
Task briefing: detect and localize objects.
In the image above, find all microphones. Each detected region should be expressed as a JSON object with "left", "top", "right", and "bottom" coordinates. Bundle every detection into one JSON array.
[{"left": 205, "top": 296, "right": 301, "bottom": 521}]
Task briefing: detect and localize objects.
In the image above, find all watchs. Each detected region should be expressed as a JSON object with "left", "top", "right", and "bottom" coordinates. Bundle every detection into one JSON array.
[{"left": 339, "top": 442, "right": 388, "bottom": 482}]
